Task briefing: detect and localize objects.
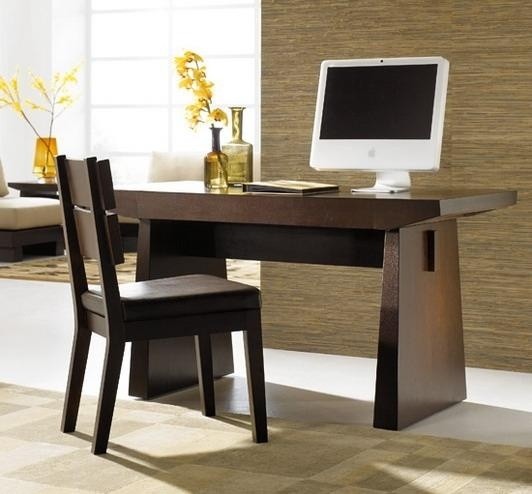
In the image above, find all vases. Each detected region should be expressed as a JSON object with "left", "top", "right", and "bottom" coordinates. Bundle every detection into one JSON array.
[
  {"left": 205, "top": 127, "right": 227, "bottom": 189},
  {"left": 32, "top": 138, "right": 58, "bottom": 179}
]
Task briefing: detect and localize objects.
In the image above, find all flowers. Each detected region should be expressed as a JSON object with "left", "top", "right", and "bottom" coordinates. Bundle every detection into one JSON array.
[
  {"left": 0, "top": 64, "right": 80, "bottom": 174},
  {"left": 175, "top": 51, "right": 227, "bottom": 184}
]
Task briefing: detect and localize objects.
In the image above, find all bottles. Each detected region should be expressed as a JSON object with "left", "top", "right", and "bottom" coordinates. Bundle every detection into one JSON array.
[{"left": 202, "top": 126, "right": 230, "bottom": 190}]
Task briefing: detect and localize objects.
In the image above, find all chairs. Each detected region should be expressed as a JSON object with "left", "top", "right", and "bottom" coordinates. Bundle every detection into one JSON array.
[
  {"left": 54, "top": 154, "right": 268, "bottom": 454},
  {"left": 0, "top": 152, "right": 64, "bottom": 262}
]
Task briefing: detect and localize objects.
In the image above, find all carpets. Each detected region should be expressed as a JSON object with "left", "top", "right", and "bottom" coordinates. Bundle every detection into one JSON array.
[
  {"left": 0, "top": 252, "right": 261, "bottom": 290},
  {"left": 0, "top": 383, "right": 532, "bottom": 494}
]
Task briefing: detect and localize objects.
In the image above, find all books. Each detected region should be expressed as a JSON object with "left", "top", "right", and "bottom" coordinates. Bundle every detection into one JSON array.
[{"left": 242, "top": 179, "right": 339, "bottom": 196}]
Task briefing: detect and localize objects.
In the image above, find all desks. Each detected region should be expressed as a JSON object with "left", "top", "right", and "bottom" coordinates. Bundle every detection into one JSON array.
[
  {"left": 8, "top": 178, "right": 58, "bottom": 197},
  {"left": 107, "top": 181, "right": 517, "bottom": 431}
]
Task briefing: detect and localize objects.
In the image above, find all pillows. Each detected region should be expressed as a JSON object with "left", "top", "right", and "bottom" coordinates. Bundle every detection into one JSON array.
[{"left": 0, "top": 155, "right": 9, "bottom": 196}]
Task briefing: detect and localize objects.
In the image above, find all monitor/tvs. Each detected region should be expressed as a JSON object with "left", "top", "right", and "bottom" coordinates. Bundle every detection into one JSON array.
[{"left": 309, "top": 56, "right": 449, "bottom": 192}]
